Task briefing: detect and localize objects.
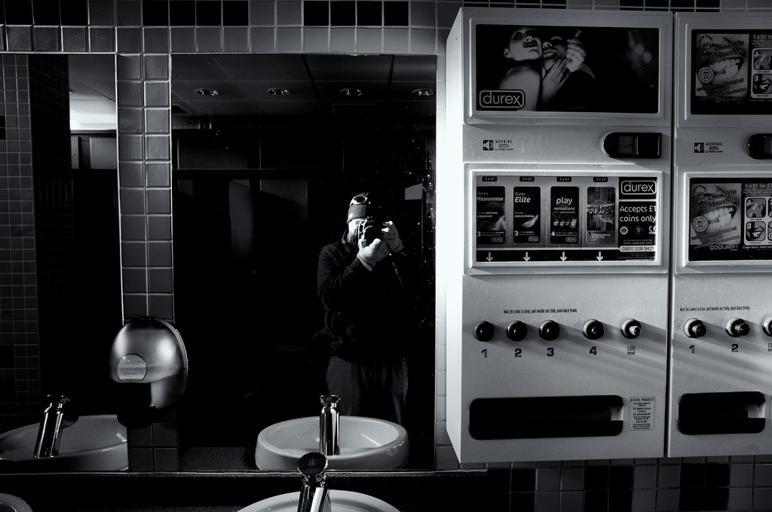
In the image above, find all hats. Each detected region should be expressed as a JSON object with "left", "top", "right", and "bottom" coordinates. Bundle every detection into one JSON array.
[{"left": 346, "top": 193, "right": 385, "bottom": 225}]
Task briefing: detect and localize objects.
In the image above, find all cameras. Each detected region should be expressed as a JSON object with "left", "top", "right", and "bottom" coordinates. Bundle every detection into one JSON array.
[{"left": 357, "top": 217, "right": 385, "bottom": 245}]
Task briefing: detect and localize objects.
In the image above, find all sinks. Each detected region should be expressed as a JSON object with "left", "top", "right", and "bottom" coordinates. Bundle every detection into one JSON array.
[
  {"left": 0, "top": 493, "right": 32, "bottom": 512},
  {"left": 0, "top": 414, "right": 129, "bottom": 472},
  {"left": 239, "top": 488, "right": 399, "bottom": 512},
  {"left": 253, "top": 415, "right": 411, "bottom": 472}
]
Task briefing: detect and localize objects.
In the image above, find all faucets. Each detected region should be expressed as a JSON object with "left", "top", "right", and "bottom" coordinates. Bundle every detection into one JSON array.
[
  {"left": 295, "top": 452, "right": 330, "bottom": 512},
  {"left": 319, "top": 392, "right": 340, "bottom": 456},
  {"left": 33, "top": 392, "right": 70, "bottom": 457}
]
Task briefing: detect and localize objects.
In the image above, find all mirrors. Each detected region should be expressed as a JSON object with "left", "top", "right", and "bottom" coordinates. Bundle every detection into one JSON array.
[
  {"left": 3, "top": 56, "right": 129, "bottom": 475},
  {"left": 168, "top": 52, "right": 436, "bottom": 478}
]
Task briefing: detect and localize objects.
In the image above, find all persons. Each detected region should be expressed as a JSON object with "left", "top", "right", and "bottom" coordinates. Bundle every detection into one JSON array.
[
  {"left": 752, "top": 74, "right": 771, "bottom": 96},
  {"left": 689, "top": 181, "right": 740, "bottom": 248},
  {"left": 693, "top": 31, "right": 749, "bottom": 98},
  {"left": 542, "top": 35, "right": 598, "bottom": 112},
  {"left": 745, "top": 198, "right": 766, "bottom": 218},
  {"left": 745, "top": 221, "right": 765, "bottom": 242},
  {"left": 491, "top": 27, "right": 587, "bottom": 112},
  {"left": 754, "top": 50, "right": 772, "bottom": 70},
  {"left": 313, "top": 191, "right": 421, "bottom": 426}
]
